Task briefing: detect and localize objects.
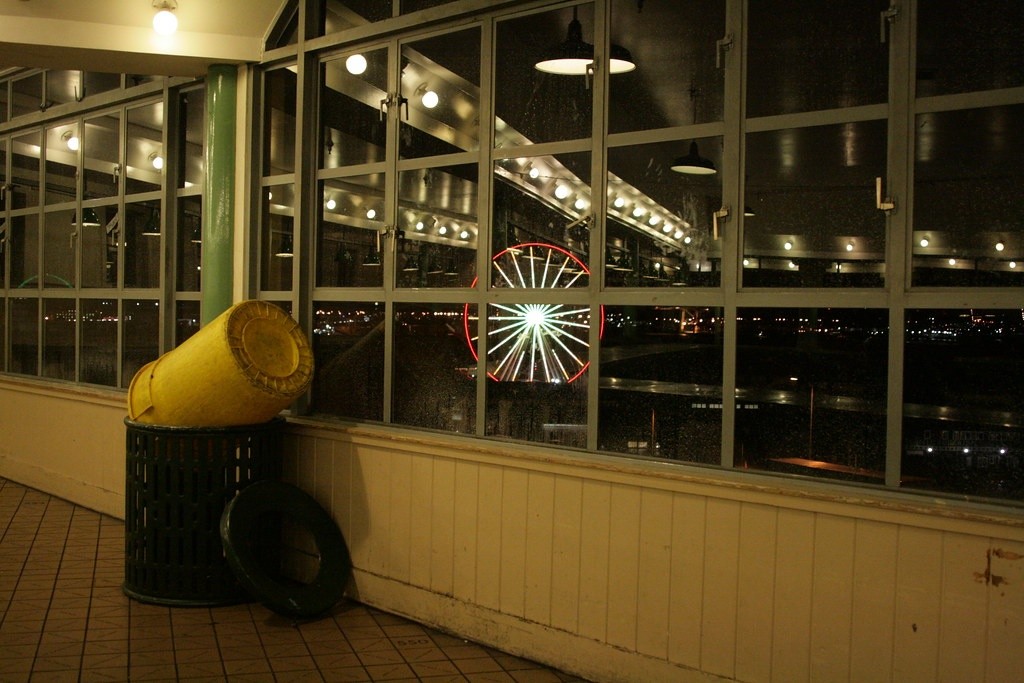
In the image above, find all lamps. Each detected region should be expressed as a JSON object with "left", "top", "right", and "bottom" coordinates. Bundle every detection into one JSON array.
[
  {"left": 151, "top": 0, "right": 179, "bottom": 37},
  {"left": 362, "top": 233, "right": 381, "bottom": 266},
  {"left": 190, "top": 198, "right": 201, "bottom": 243},
  {"left": 141, "top": 202, "right": 161, "bottom": 236},
  {"left": 274, "top": 235, "right": 293, "bottom": 257},
  {"left": 402, "top": 244, "right": 419, "bottom": 271},
  {"left": 61, "top": 130, "right": 78, "bottom": 150},
  {"left": 445, "top": 250, "right": 460, "bottom": 275},
  {"left": 414, "top": 80, "right": 438, "bottom": 108},
  {"left": 426, "top": 258, "right": 444, "bottom": 274},
  {"left": 346, "top": 54, "right": 368, "bottom": 75},
  {"left": 70, "top": 172, "right": 101, "bottom": 227},
  {"left": 744, "top": 203, "right": 756, "bottom": 217},
  {"left": 334, "top": 229, "right": 354, "bottom": 265},
  {"left": 533, "top": 4, "right": 593, "bottom": 75},
  {"left": 669, "top": 87, "right": 717, "bottom": 175},
  {"left": 609, "top": 42, "right": 636, "bottom": 74},
  {"left": 148, "top": 152, "right": 163, "bottom": 170}
]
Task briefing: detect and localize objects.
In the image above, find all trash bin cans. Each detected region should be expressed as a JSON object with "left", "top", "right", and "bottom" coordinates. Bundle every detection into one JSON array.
[
  {"left": 121, "top": 414, "right": 286, "bottom": 608},
  {"left": 128, "top": 300, "right": 315, "bottom": 460}
]
